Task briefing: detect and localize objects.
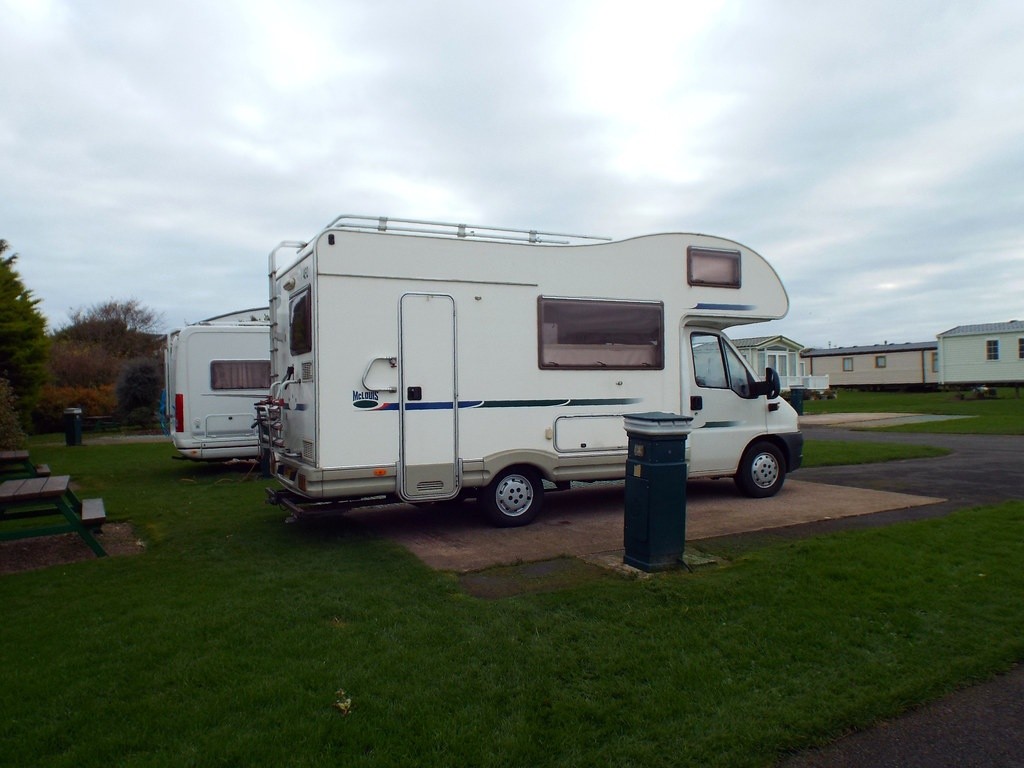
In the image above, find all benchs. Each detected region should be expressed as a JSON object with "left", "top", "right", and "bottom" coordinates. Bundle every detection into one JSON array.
[
  {"left": 81, "top": 497, "right": 107, "bottom": 528},
  {"left": 34, "top": 463, "right": 53, "bottom": 477}
]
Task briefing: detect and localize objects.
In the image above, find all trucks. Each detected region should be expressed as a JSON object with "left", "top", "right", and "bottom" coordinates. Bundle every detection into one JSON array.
[
  {"left": 164, "top": 322, "right": 277, "bottom": 464},
  {"left": 250, "top": 211, "right": 805, "bottom": 528}
]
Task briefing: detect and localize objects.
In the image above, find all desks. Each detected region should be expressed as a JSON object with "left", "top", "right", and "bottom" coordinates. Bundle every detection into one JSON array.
[
  {"left": 0, "top": 450, "right": 36, "bottom": 474},
  {"left": 0, "top": 472, "right": 108, "bottom": 560}
]
